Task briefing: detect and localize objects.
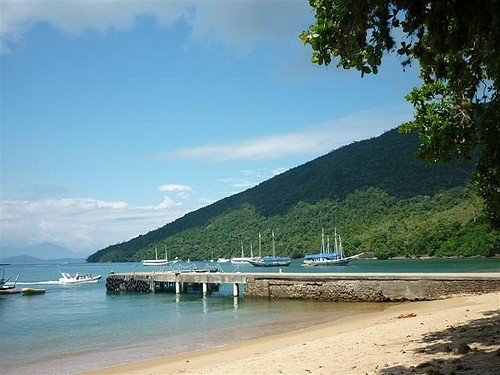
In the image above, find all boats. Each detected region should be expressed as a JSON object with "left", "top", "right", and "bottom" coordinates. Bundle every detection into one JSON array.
[
  {"left": 231, "top": 231, "right": 291, "bottom": 267},
  {"left": 141, "top": 248, "right": 169, "bottom": 265},
  {"left": 0, "top": 268, "right": 19, "bottom": 290},
  {"left": 300, "top": 228, "right": 364, "bottom": 266},
  {"left": 217, "top": 257, "right": 230, "bottom": 262},
  {"left": 59, "top": 272, "right": 101, "bottom": 283}
]
